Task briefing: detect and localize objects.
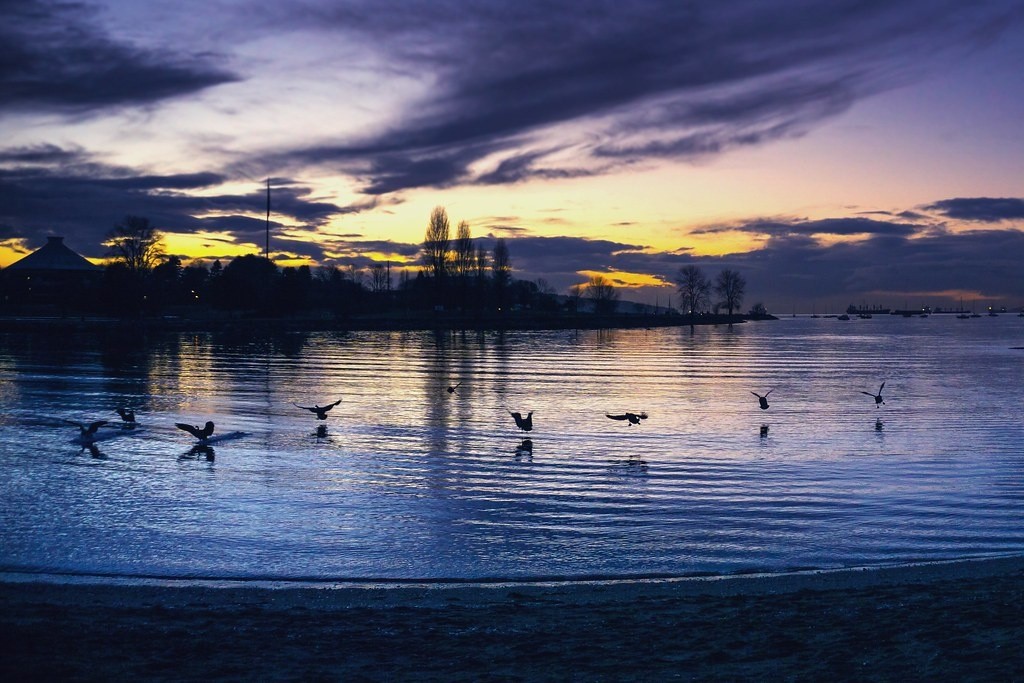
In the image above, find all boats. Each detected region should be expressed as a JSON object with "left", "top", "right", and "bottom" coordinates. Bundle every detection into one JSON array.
[
  {"left": 957, "top": 314, "right": 969, "bottom": 318},
  {"left": 971, "top": 314, "right": 981, "bottom": 317},
  {"left": 823, "top": 315, "right": 837, "bottom": 318},
  {"left": 838, "top": 314, "right": 850, "bottom": 320},
  {"left": 920, "top": 314, "right": 928, "bottom": 318},
  {"left": 903, "top": 314, "right": 911, "bottom": 317},
  {"left": 811, "top": 315, "right": 820, "bottom": 318},
  {"left": 1017, "top": 313, "right": 1024, "bottom": 316},
  {"left": 989, "top": 313, "right": 998, "bottom": 316}
]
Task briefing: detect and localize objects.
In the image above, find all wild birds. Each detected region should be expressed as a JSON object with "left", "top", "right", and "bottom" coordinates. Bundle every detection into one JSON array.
[
  {"left": 605, "top": 411, "right": 648, "bottom": 427},
  {"left": 758, "top": 424, "right": 769, "bottom": 441},
  {"left": 174, "top": 420, "right": 214, "bottom": 441},
  {"left": 873, "top": 417, "right": 884, "bottom": 435},
  {"left": 310, "top": 424, "right": 340, "bottom": 444},
  {"left": 860, "top": 381, "right": 886, "bottom": 408},
  {"left": 513, "top": 438, "right": 533, "bottom": 460},
  {"left": 76, "top": 441, "right": 104, "bottom": 459},
  {"left": 115, "top": 408, "right": 135, "bottom": 424},
  {"left": 174, "top": 444, "right": 216, "bottom": 464},
  {"left": 292, "top": 399, "right": 342, "bottom": 420},
  {"left": 506, "top": 408, "right": 534, "bottom": 434},
  {"left": 750, "top": 389, "right": 774, "bottom": 410},
  {"left": 65, "top": 420, "right": 109, "bottom": 437}
]
